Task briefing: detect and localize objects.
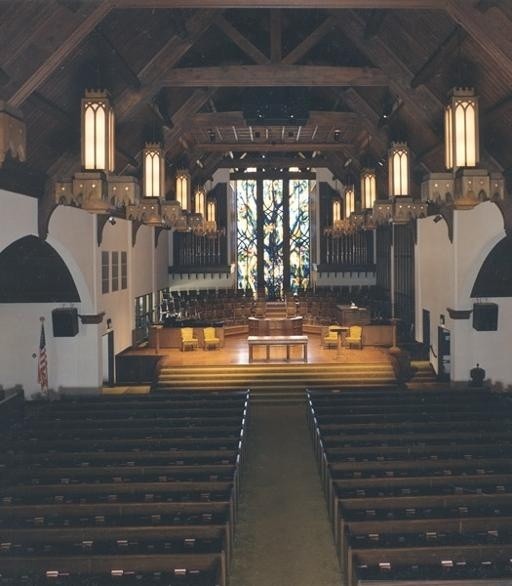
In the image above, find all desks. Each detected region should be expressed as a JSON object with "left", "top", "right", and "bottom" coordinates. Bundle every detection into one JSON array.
[{"left": 247, "top": 335, "right": 309, "bottom": 364}]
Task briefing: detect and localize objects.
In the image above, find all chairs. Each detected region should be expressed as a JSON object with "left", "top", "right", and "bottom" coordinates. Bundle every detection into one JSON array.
[
  {"left": 160, "top": 289, "right": 265, "bottom": 320},
  {"left": 283, "top": 288, "right": 338, "bottom": 323},
  {"left": 345, "top": 324, "right": 364, "bottom": 350},
  {"left": 324, "top": 324, "right": 341, "bottom": 351},
  {"left": 202, "top": 327, "right": 220, "bottom": 350},
  {"left": 179, "top": 326, "right": 199, "bottom": 352}
]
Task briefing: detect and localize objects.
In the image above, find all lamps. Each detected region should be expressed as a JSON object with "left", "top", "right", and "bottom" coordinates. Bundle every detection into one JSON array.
[
  {"left": 124, "top": 142, "right": 180, "bottom": 229},
  {"left": 323, "top": 200, "right": 343, "bottom": 239},
  {"left": 194, "top": 184, "right": 208, "bottom": 233},
  {"left": 53, "top": 87, "right": 139, "bottom": 213},
  {"left": 372, "top": 141, "right": 427, "bottom": 223},
  {"left": 419, "top": 86, "right": 506, "bottom": 208},
  {"left": 336, "top": 186, "right": 362, "bottom": 234},
  {"left": 172, "top": 167, "right": 202, "bottom": 234},
  {"left": 205, "top": 200, "right": 220, "bottom": 234},
  {"left": 350, "top": 172, "right": 383, "bottom": 230}
]
